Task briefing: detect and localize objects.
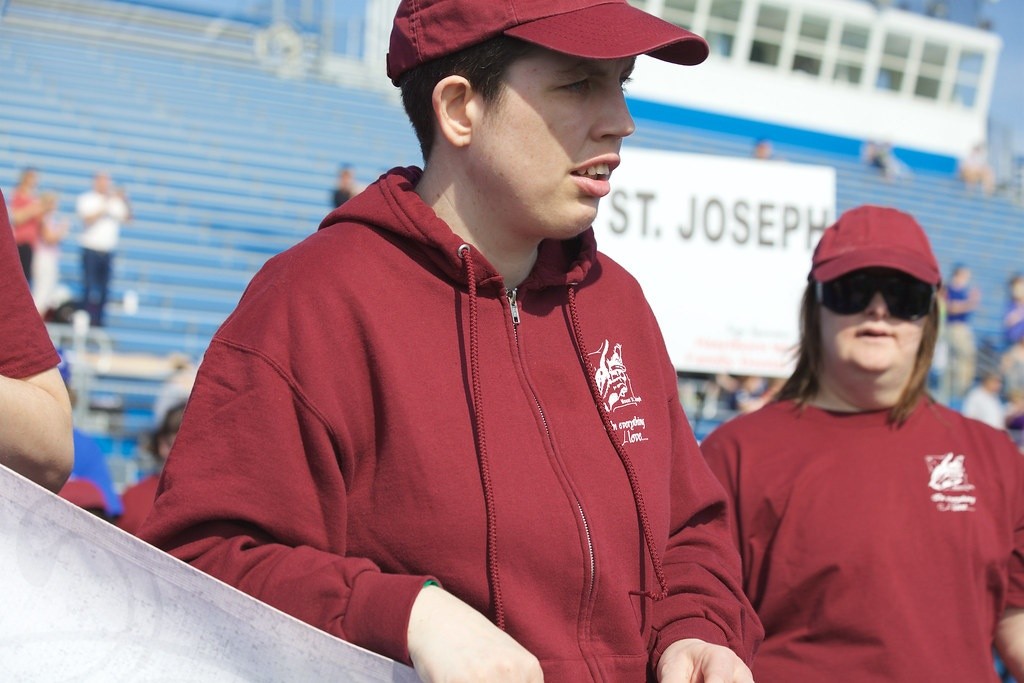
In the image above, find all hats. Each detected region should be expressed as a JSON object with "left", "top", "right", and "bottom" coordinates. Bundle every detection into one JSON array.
[
  {"left": 807, "top": 205, "right": 941, "bottom": 290},
  {"left": 386, "top": 0, "right": 709, "bottom": 88}
]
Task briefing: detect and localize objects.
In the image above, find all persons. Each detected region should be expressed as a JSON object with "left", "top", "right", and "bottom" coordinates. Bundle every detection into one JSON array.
[
  {"left": 74, "top": 172, "right": 132, "bottom": 327},
  {"left": 943, "top": 259, "right": 980, "bottom": 395},
  {"left": 751, "top": 138, "right": 773, "bottom": 158},
  {"left": 1, "top": 190, "right": 77, "bottom": 494},
  {"left": 329, "top": 163, "right": 356, "bottom": 209},
  {"left": 1004, "top": 275, "right": 1024, "bottom": 423},
  {"left": 961, "top": 372, "right": 1006, "bottom": 429},
  {"left": 7, "top": 166, "right": 74, "bottom": 318},
  {"left": 139, "top": 0, "right": 765, "bottom": 683},
  {"left": 957, "top": 143, "right": 996, "bottom": 194},
  {"left": 861, "top": 140, "right": 914, "bottom": 186},
  {"left": 696, "top": 207, "right": 1024, "bottom": 683},
  {"left": 731, "top": 376, "right": 782, "bottom": 411},
  {"left": 117, "top": 361, "right": 197, "bottom": 539}
]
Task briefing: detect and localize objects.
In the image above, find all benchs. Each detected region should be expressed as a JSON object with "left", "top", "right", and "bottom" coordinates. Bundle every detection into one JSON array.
[{"left": 0, "top": 0, "right": 1024, "bottom": 488}]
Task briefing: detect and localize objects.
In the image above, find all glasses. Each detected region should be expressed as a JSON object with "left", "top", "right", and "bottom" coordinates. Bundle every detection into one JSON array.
[{"left": 813, "top": 267, "right": 937, "bottom": 322}]
